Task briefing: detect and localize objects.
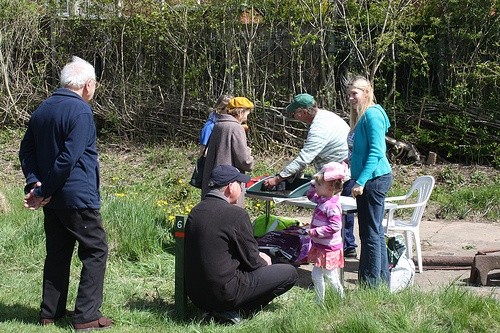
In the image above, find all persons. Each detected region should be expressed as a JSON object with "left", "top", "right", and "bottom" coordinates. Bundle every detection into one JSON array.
[
  {"left": 181, "top": 164, "right": 298, "bottom": 324},
  {"left": 345, "top": 77, "right": 393, "bottom": 292},
  {"left": 305, "top": 162, "right": 349, "bottom": 307},
  {"left": 263, "top": 94, "right": 358, "bottom": 259},
  {"left": 18, "top": 56, "right": 112, "bottom": 331},
  {"left": 200, "top": 95, "right": 254, "bottom": 209}
]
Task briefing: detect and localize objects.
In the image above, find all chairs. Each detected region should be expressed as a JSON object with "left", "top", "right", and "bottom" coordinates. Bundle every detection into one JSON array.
[{"left": 382, "top": 174, "right": 436, "bottom": 274}]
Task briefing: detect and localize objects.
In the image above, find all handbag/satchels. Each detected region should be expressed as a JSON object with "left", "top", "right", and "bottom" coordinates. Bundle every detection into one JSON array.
[
  {"left": 252, "top": 214, "right": 295, "bottom": 237},
  {"left": 384, "top": 234, "right": 415, "bottom": 294},
  {"left": 189, "top": 157, "right": 206, "bottom": 189}
]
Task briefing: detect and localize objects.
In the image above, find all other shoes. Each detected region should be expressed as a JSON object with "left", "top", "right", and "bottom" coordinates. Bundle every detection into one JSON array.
[
  {"left": 223, "top": 311, "right": 241, "bottom": 324},
  {"left": 74, "top": 317, "right": 112, "bottom": 331},
  {"left": 344, "top": 249, "right": 357, "bottom": 257},
  {"left": 40, "top": 311, "right": 74, "bottom": 325}
]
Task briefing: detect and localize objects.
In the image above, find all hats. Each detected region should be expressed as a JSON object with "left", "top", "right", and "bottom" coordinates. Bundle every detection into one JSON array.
[
  {"left": 228, "top": 97, "right": 253, "bottom": 109},
  {"left": 286, "top": 94, "right": 314, "bottom": 112},
  {"left": 207, "top": 165, "right": 251, "bottom": 190}
]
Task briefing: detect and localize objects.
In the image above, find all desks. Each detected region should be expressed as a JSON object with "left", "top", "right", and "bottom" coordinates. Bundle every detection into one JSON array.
[{"left": 244, "top": 187, "right": 398, "bottom": 287}]
